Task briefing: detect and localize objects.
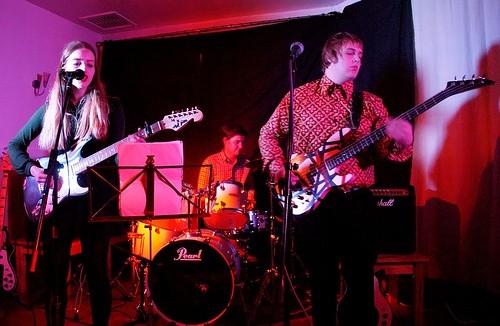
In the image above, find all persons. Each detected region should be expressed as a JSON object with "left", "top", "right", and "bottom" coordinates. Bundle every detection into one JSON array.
[
  {"left": 7, "top": 39, "right": 147, "bottom": 326},
  {"left": 198, "top": 124, "right": 269, "bottom": 279},
  {"left": 259, "top": 32, "right": 412, "bottom": 326}
]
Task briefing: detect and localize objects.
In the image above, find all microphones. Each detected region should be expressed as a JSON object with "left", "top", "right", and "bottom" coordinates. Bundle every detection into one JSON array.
[
  {"left": 62, "top": 69, "right": 85, "bottom": 80},
  {"left": 200, "top": 283, "right": 208, "bottom": 293},
  {"left": 289, "top": 42, "right": 304, "bottom": 61}
]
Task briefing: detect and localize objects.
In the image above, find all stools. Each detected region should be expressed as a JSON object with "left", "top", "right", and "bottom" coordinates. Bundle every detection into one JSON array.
[
  {"left": 10, "top": 237, "right": 82, "bottom": 299},
  {"left": 373, "top": 251, "right": 433, "bottom": 326}
]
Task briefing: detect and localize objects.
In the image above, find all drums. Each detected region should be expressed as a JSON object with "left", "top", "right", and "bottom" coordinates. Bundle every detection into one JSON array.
[
  {"left": 145, "top": 229, "right": 240, "bottom": 326},
  {"left": 203, "top": 178, "right": 249, "bottom": 230},
  {"left": 226, "top": 210, "right": 270, "bottom": 238},
  {"left": 130, "top": 219, "right": 182, "bottom": 261}
]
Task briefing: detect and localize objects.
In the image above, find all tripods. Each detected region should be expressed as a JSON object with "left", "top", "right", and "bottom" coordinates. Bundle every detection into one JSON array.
[{"left": 247, "top": 176, "right": 306, "bottom": 326}]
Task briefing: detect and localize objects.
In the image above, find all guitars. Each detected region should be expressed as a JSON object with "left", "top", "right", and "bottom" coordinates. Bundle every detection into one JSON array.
[
  {"left": 0, "top": 151, "right": 19, "bottom": 292},
  {"left": 22, "top": 105, "right": 205, "bottom": 223},
  {"left": 275, "top": 74, "right": 495, "bottom": 217}
]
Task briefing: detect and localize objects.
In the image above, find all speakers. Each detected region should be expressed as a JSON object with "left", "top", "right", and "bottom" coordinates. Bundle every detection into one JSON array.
[{"left": 363, "top": 185, "right": 416, "bottom": 255}]
